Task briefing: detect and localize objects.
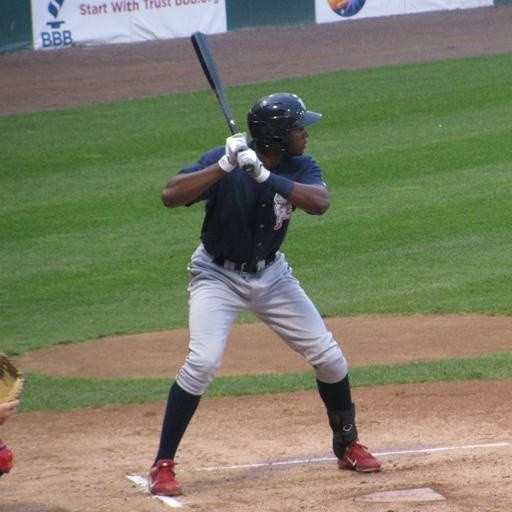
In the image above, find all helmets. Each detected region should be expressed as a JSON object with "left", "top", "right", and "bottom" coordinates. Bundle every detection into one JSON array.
[{"left": 247, "top": 93, "right": 322, "bottom": 154}]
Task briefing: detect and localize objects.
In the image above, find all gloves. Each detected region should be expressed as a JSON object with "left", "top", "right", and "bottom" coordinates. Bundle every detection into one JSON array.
[{"left": 218, "top": 132, "right": 270, "bottom": 183}]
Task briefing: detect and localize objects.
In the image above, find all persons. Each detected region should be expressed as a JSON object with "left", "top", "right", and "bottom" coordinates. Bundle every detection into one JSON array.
[
  {"left": 146, "top": 92, "right": 384, "bottom": 498},
  {"left": 0, "top": 398, "right": 21, "bottom": 477}
]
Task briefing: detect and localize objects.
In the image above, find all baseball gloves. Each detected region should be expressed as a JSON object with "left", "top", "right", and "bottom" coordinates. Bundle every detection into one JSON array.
[{"left": 0, "top": 352, "right": 26, "bottom": 403}]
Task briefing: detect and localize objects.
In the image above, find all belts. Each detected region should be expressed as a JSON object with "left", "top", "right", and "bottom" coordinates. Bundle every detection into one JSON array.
[{"left": 211, "top": 256, "right": 275, "bottom": 272}]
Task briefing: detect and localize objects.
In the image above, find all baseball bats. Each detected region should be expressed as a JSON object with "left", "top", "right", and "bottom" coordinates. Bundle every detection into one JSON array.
[{"left": 189, "top": 32, "right": 254, "bottom": 173}]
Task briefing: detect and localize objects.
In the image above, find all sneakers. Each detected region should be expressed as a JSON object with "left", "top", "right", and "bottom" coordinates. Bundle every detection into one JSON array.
[
  {"left": 149, "top": 458, "right": 181, "bottom": 495},
  {"left": 338, "top": 438, "right": 380, "bottom": 471}
]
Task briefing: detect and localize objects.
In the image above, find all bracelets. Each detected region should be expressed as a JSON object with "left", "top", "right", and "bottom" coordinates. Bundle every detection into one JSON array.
[{"left": 262, "top": 172, "right": 295, "bottom": 198}]
